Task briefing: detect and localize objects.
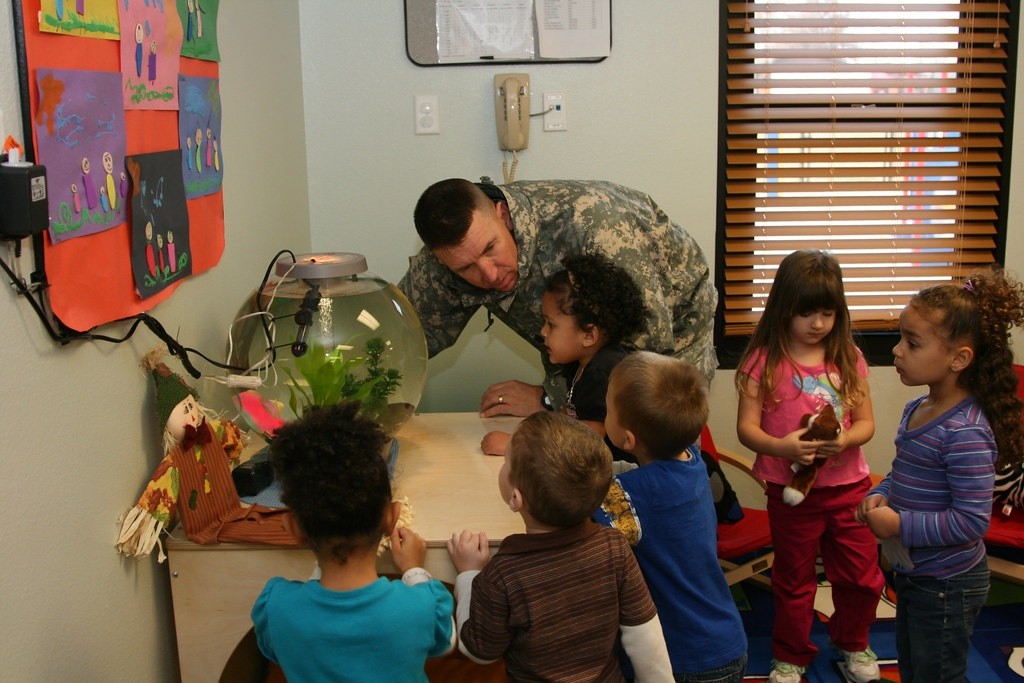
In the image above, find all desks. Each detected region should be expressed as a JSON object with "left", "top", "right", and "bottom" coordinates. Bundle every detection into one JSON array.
[{"left": 168, "top": 410, "right": 530, "bottom": 682}]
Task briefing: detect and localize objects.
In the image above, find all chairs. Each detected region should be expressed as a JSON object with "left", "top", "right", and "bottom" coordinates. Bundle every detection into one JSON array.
[
  {"left": 979, "top": 364, "right": 1024, "bottom": 582},
  {"left": 699, "top": 422, "right": 783, "bottom": 592}
]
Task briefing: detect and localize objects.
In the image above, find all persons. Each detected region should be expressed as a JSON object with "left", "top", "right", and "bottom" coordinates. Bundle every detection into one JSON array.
[
  {"left": 392, "top": 179, "right": 719, "bottom": 464},
  {"left": 446, "top": 409, "right": 675, "bottom": 683},
  {"left": 480, "top": 253, "right": 651, "bottom": 467},
  {"left": 732, "top": 250, "right": 886, "bottom": 683},
  {"left": 120, "top": 357, "right": 416, "bottom": 566},
  {"left": 854, "top": 268, "right": 1024, "bottom": 683},
  {"left": 589, "top": 350, "right": 749, "bottom": 683},
  {"left": 250, "top": 403, "right": 459, "bottom": 682}
]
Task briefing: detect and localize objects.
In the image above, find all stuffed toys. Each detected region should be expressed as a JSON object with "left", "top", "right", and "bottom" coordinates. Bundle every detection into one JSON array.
[{"left": 781, "top": 403, "right": 845, "bottom": 507}]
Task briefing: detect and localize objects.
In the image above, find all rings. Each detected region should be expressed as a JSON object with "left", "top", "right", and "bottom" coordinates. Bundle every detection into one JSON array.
[{"left": 497, "top": 394, "right": 504, "bottom": 405}]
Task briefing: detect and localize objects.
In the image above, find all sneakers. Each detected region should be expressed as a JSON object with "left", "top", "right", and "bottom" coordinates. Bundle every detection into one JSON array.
[
  {"left": 840, "top": 645, "right": 881, "bottom": 683},
  {"left": 769, "top": 658, "right": 806, "bottom": 683}
]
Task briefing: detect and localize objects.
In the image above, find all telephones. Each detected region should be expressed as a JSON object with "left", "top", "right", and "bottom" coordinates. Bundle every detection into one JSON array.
[{"left": 493, "top": 71, "right": 532, "bottom": 152}]
